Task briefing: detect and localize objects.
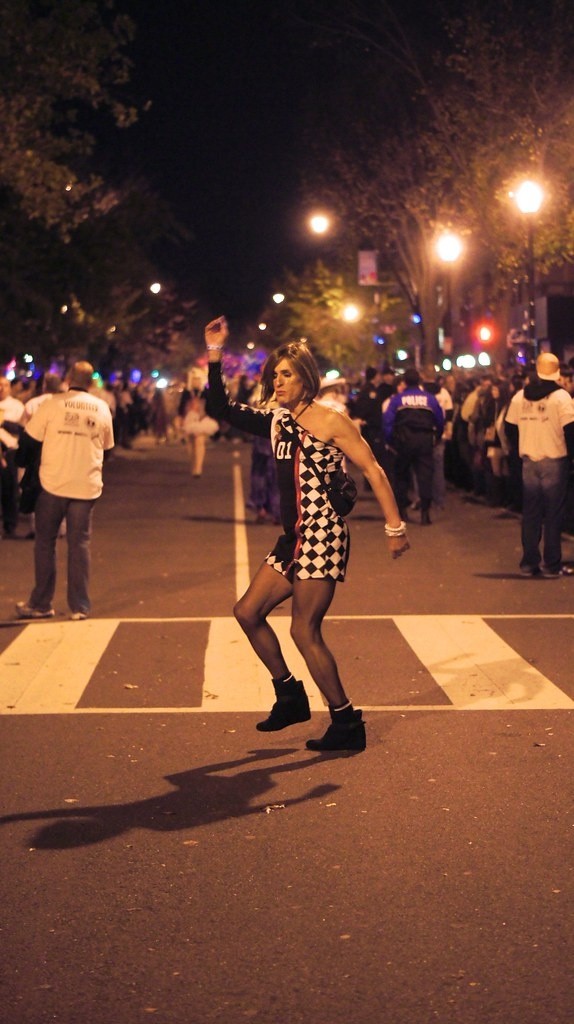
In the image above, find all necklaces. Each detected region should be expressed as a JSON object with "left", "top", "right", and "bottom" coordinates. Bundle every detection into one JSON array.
[{"left": 293, "top": 400, "right": 312, "bottom": 421}]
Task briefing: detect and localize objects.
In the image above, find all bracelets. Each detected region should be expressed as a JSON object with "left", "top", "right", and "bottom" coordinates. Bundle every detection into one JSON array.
[
  {"left": 385, "top": 528, "right": 406, "bottom": 537},
  {"left": 385, "top": 521, "right": 405, "bottom": 531},
  {"left": 207, "top": 345, "right": 224, "bottom": 351}
]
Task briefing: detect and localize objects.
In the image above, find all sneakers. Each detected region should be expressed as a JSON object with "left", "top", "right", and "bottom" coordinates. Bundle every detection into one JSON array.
[
  {"left": 16, "top": 601, "right": 55, "bottom": 617},
  {"left": 58, "top": 610, "right": 89, "bottom": 620}
]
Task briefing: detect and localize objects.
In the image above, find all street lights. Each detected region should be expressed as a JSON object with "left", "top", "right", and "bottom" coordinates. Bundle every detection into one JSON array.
[
  {"left": 436, "top": 225, "right": 463, "bottom": 363},
  {"left": 515, "top": 180, "right": 544, "bottom": 359}
]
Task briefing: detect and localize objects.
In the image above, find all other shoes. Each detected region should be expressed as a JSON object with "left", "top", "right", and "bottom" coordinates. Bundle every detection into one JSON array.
[
  {"left": 519, "top": 564, "right": 540, "bottom": 576},
  {"left": 271, "top": 515, "right": 280, "bottom": 524},
  {"left": 256, "top": 508, "right": 266, "bottom": 524},
  {"left": 541, "top": 562, "right": 574, "bottom": 577},
  {"left": 420, "top": 510, "right": 431, "bottom": 525},
  {"left": 399, "top": 511, "right": 409, "bottom": 522}
]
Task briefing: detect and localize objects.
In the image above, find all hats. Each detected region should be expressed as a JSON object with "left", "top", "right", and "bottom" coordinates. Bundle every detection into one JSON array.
[{"left": 536, "top": 353, "right": 560, "bottom": 380}]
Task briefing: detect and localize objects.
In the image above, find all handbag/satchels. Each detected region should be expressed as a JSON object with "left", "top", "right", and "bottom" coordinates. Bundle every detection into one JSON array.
[
  {"left": 326, "top": 473, "right": 358, "bottom": 517},
  {"left": 485, "top": 426, "right": 496, "bottom": 441}
]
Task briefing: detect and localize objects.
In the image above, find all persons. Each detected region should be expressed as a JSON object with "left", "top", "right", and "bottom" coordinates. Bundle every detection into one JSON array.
[
  {"left": 15, "top": 361, "right": 113, "bottom": 619},
  {"left": 382, "top": 369, "right": 444, "bottom": 526},
  {"left": 505, "top": 353, "right": 574, "bottom": 577},
  {"left": 0, "top": 358, "right": 573, "bottom": 540},
  {"left": 204, "top": 316, "right": 410, "bottom": 753}
]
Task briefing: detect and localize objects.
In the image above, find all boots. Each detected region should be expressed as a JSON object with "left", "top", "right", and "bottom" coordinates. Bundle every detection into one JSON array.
[
  {"left": 256, "top": 679, "right": 311, "bottom": 732},
  {"left": 306, "top": 705, "right": 366, "bottom": 751}
]
